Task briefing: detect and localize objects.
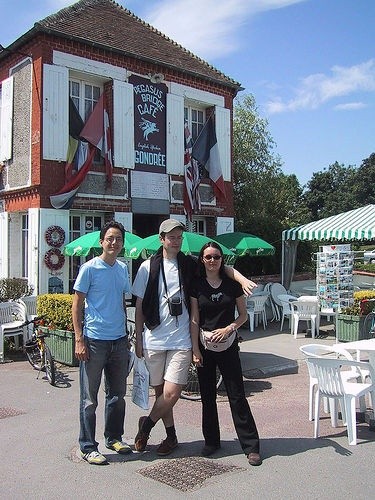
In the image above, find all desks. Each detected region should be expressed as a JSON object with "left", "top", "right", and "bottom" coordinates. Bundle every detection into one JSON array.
[
  {"left": 297, "top": 296, "right": 333, "bottom": 329},
  {"left": 333, "top": 337, "right": 375, "bottom": 407}
]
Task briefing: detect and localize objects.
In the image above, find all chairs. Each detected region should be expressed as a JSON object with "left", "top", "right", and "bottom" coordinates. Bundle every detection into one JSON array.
[
  {"left": 0, "top": 295, "right": 37, "bottom": 364},
  {"left": 235, "top": 282, "right": 375, "bottom": 446}
]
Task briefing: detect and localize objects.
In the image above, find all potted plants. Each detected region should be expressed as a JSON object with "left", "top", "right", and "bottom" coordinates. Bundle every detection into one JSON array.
[
  {"left": 36, "top": 293, "right": 85, "bottom": 367},
  {"left": 336, "top": 289, "right": 375, "bottom": 343}
]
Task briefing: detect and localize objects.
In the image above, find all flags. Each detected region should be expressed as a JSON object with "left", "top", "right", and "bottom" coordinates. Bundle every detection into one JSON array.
[
  {"left": 184, "top": 109, "right": 227, "bottom": 223},
  {"left": 46, "top": 92, "right": 113, "bottom": 209}
]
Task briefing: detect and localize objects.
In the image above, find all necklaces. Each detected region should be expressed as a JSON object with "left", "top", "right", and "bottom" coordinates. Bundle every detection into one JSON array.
[{"left": 207, "top": 278, "right": 222, "bottom": 288}]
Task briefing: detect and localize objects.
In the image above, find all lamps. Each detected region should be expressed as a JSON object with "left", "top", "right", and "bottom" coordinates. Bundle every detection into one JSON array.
[{"left": 150, "top": 72, "right": 164, "bottom": 85}]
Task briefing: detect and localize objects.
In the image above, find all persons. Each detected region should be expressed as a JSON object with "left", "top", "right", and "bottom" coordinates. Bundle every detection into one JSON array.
[
  {"left": 190, "top": 241, "right": 262, "bottom": 466},
  {"left": 131, "top": 219, "right": 259, "bottom": 456},
  {"left": 72, "top": 221, "right": 131, "bottom": 465}
]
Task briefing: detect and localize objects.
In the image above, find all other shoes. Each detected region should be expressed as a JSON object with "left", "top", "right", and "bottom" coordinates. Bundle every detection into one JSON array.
[
  {"left": 248, "top": 452, "right": 261, "bottom": 465},
  {"left": 201, "top": 443, "right": 221, "bottom": 456}
]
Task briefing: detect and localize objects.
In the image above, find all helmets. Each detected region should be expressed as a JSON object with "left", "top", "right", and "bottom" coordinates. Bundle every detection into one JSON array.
[{"left": 24, "top": 341, "right": 38, "bottom": 353}]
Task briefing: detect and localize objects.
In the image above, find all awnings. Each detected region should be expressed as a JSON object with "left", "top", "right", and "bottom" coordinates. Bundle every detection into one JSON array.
[
  {"left": 212, "top": 232, "right": 275, "bottom": 256},
  {"left": 61, "top": 229, "right": 144, "bottom": 258},
  {"left": 130, "top": 232, "right": 238, "bottom": 265}
]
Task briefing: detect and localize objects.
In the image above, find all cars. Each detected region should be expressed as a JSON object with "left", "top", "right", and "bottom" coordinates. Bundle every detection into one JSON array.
[{"left": 363, "top": 248, "right": 375, "bottom": 265}]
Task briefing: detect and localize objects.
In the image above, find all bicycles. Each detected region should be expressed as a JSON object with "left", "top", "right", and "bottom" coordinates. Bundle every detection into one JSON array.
[
  {"left": 18, "top": 313, "right": 57, "bottom": 385},
  {"left": 124, "top": 323, "right": 224, "bottom": 402},
  {"left": 361, "top": 299, "right": 375, "bottom": 339}
]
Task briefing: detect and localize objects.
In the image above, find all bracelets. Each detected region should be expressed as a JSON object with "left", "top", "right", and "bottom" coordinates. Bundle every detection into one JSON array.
[{"left": 229, "top": 324, "right": 238, "bottom": 332}]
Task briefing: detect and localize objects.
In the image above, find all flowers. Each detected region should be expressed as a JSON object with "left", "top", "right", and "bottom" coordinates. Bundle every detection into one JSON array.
[{"left": 353, "top": 290, "right": 375, "bottom": 315}]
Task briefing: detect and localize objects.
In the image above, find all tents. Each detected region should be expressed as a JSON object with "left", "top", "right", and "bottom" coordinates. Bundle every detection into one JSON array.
[{"left": 281, "top": 204, "right": 375, "bottom": 290}]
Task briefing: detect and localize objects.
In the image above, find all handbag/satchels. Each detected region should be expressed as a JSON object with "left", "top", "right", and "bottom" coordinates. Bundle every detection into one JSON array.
[
  {"left": 130, "top": 356, "right": 149, "bottom": 409},
  {"left": 198, "top": 327, "right": 236, "bottom": 352},
  {"left": 168, "top": 298, "right": 183, "bottom": 316}
]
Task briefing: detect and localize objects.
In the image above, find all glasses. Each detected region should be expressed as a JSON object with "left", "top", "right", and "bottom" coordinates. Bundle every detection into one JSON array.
[{"left": 202, "top": 255, "right": 222, "bottom": 260}]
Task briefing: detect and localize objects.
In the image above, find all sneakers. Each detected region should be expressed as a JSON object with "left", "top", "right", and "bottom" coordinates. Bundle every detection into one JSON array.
[
  {"left": 135, "top": 415, "right": 150, "bottom": 451},
  {"left": 80, "top": 448, "right": 107, "bottom": 465},
  {"left": 105, "top": 439, "right": 132, "bottom": 453},
  {"left": 156, "top": 436, "right": 178, "bottom": 454}
]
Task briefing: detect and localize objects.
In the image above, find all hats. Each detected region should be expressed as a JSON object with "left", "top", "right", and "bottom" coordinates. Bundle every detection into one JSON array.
[{"left": 159, "top": 219, "right": 185, "bottom": 235}]
